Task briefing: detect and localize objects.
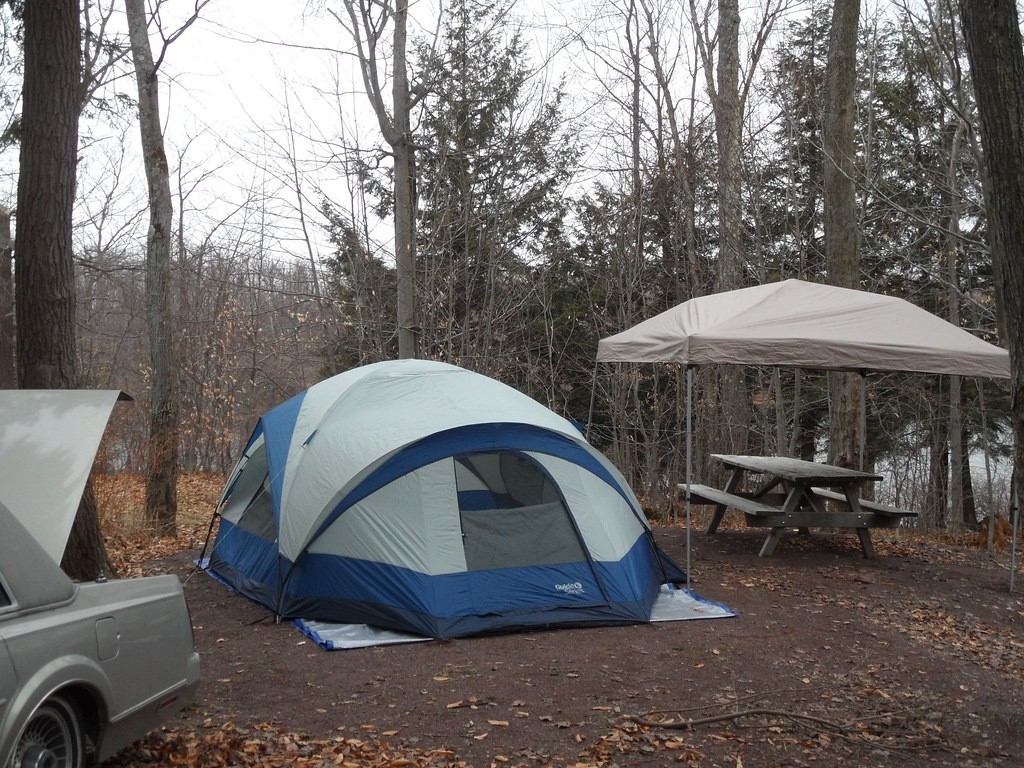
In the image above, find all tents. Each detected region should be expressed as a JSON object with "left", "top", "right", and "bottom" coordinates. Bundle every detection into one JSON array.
[
  {"left": 595, "top": 279, "right": 1018, "bottom": 595},
  {"left": 197, "top": 358, "right": 693, "bottom": 641}
]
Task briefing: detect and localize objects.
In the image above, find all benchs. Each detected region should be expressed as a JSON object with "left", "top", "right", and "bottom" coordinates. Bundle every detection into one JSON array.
[
  {"left": 810, "top": 487, "right": 919, "bottom": 518},
  {"left": 677, "top": 483, "right": 786, "bottom": 516}
]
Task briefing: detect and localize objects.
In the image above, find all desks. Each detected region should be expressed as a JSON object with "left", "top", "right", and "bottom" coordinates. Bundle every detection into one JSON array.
[{"left": 705, "top": 454, "right": 883, "bottom": 560}]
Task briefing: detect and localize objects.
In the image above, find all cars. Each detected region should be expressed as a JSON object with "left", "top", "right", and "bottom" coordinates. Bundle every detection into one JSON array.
[{"left": 0, "top": 390, "right": 202, "bottom": 768}]
